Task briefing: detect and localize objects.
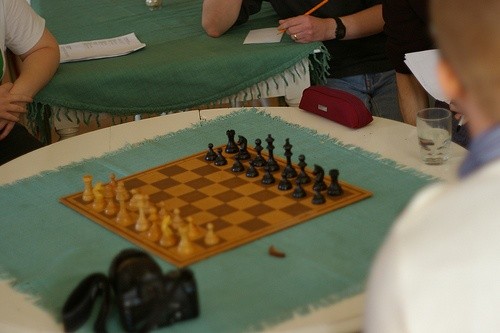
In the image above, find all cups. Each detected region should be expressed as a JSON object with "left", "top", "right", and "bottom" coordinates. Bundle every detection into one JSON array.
[
  {"left": 146, "top": 0, "right": 162, "bottom": 10},
  {"left": 416, "top": 108, "right": 452, "bottom": 165}
]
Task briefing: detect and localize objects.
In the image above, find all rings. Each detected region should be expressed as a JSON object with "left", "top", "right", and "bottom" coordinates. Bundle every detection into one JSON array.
[{"left": 292, "top": 32, "right": 298, "bottom": 42}]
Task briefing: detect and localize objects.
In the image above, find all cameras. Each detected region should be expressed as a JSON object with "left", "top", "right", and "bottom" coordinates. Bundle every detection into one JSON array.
[{"left": 108, "top": 249, "right": 199, "bottom": 333}]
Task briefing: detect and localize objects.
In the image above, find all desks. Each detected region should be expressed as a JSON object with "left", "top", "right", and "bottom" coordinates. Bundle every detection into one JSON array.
[
  {"left": 1, "top": 0, "right": 331, "bottom": 141},
  {"left": 0, "top": 107, "right": 471, "bottom": 333}
]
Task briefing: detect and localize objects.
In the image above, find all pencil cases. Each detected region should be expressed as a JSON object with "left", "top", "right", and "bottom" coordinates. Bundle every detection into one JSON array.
[{"left": 298, "top": 86, "right": 373, "bottom": 129}]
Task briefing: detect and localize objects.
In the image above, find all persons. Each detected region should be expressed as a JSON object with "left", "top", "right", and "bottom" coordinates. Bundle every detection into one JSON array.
[
  {"left": 201, "top": 0, "right": 401, "bottom": 126},
  {"left": 355, "top": 0, "right": 500, "bottom": 333},
  {"left": 0, "top": 0, "right": 60, "bottom": 167},
  {"left": 381, "top": 0, "right": 469, "bottom": 148}
]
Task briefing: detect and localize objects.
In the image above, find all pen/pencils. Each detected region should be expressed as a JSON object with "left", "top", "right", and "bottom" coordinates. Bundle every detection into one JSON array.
[{"left": 276, "top": 0, "right": 328, "bottom": 35}]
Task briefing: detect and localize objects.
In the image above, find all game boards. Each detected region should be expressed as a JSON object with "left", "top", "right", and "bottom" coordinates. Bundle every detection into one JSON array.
[{"left": 60, "top": 140, "right": 372, "bottom": 270}]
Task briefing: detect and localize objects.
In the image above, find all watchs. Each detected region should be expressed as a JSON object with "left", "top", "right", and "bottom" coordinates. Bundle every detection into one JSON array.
[{"left": 331, "top": 16, "right": 346, "bottom": 42}]
